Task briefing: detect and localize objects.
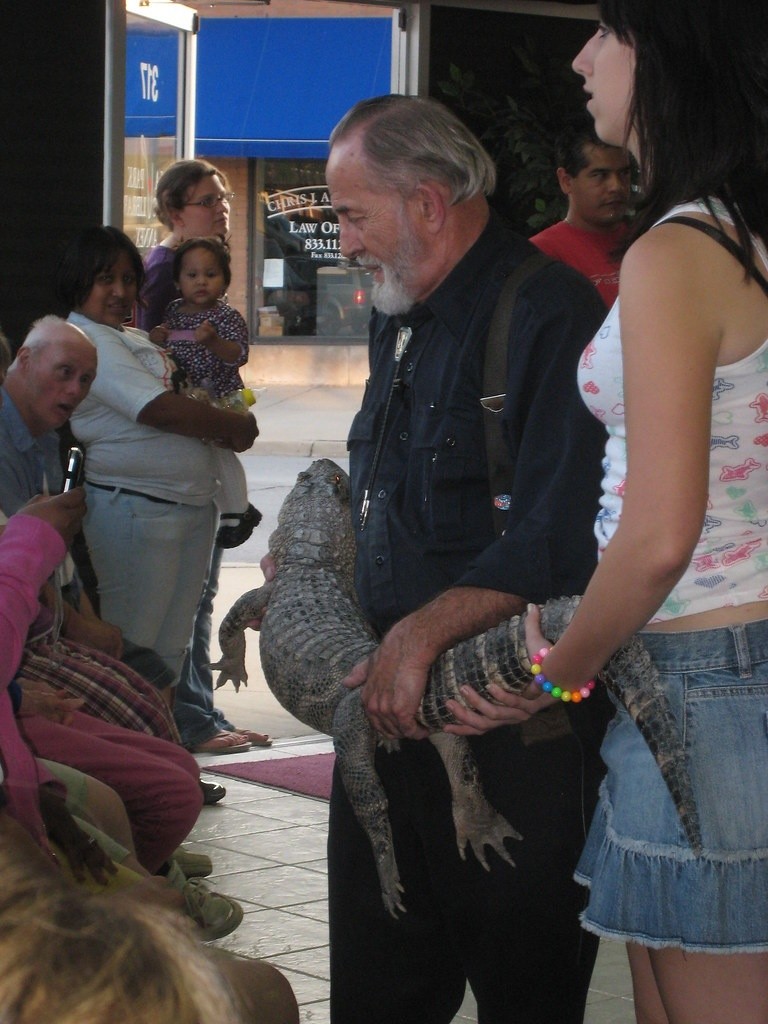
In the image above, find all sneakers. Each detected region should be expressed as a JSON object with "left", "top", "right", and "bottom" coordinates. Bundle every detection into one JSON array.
[
  {"left": 171, "top": 848, "right": 213, "bottom": 877},
  {"left": 180, "top": 879, "right": 244, "bottom": 942}
]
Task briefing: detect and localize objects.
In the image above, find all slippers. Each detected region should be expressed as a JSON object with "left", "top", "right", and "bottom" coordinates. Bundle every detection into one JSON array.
[
  {"left": 227, "top": 727, "right": 273, "bottom": 745},
  {"left": 188, "top": 728, "right": 252, "bottom": 753}
]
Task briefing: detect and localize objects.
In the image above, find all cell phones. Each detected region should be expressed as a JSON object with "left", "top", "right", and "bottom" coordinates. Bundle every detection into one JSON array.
[{"left": 62, "top": 449, "right": 84, "bottom": 493}]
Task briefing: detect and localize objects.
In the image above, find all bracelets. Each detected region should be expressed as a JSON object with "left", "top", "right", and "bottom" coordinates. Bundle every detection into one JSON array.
[{"left": 528, "top": 646, "right": 595, "bottom": 704}]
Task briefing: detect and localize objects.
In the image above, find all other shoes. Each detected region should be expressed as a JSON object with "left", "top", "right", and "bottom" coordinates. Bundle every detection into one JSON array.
[
  {"left": 216, "top": 503, "right": 261, "bottom": 549},
  {"left": 197, "top": 778, "right": 226, "bottom": 802}
]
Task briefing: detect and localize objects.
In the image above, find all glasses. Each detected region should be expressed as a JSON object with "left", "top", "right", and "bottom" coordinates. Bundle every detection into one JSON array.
[{"left": 180, "top": 192, "right": 235, "bottom": 209}]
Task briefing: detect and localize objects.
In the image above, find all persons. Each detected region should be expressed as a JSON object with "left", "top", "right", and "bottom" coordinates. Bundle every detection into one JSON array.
[
  {"left": 524, "top": 0, "right": 768, "bottom": 1024},
  {"left": 0, "top": 156, "right": 300, "bottom": 1024},
  {"left": 529, "top": 127, "right": 634, "bottom": 312},
  {"left": 256, "top": 96, "right": 602, "bottom": 1024}
]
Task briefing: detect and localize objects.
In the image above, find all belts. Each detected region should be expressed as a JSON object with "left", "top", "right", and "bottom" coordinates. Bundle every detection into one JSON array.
[{"left": 86, "top": 478, "right": 180, "bottom": 505}]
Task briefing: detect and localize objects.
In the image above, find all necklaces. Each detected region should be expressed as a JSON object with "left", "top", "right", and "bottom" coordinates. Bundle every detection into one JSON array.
[{"left": 564, "top": 216, "right": 572, "bottom": 224}]
